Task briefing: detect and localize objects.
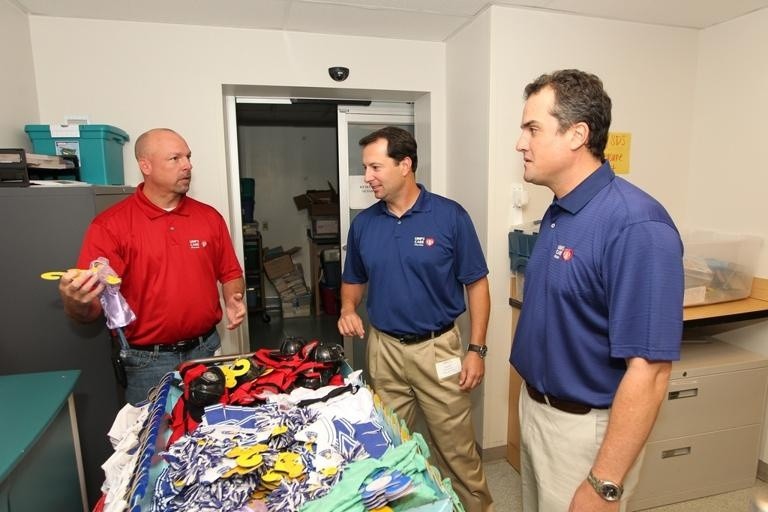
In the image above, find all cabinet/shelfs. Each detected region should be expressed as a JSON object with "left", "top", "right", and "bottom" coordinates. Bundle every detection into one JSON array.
[
  {"left": 242, "top": 232, "right": 268, "bottom": 322},
  {"left": 0, "top": 367, "right": 96, "bottom": 512},
  {"left": 308, "top": 239, "right": 342, "bottom": 317},
  {"left": 618, "top": 323, "right": 768, "bottom": 512},
  {"left": 0, "top": 183, "right": 141, "bottom": 512}
]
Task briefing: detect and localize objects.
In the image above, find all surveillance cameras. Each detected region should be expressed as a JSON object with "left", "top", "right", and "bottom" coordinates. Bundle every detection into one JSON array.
[{"left": 329, "top": 67, "right": 349, "bottom": 81}]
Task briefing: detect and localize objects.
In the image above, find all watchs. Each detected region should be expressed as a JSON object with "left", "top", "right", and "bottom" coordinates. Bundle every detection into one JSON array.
[
  {"left": 586, "top": 473, "right": 625, "bottom": 502},
  {"left": 467, "top": 344, "right": 488, "bottom": 358}
]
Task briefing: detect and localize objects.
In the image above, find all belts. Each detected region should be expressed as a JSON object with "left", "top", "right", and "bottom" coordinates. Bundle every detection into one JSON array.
[
  {"left": 527, "top": 380, "right": 609, "bottom": 414},
  {"left": 130, "top": 325, "right": 216, "bottom": 353},
  {"left": 381, "top": 321, "right": 454, "bottom": 345}
]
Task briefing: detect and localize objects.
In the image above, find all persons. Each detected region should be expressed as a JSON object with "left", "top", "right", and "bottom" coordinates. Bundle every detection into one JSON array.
[
  {"left": 508, "top": 70, "right": 685, "bottom": 512},
  {"left": 59, "top": 129, "right": 246, "bottom": 414},
  {"left": 334, "top": 127, "right": 496, "bottom": 511}
]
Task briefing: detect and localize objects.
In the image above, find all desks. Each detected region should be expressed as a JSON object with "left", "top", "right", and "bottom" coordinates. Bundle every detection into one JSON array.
[{"left": 506, "top": 258, "right": 768, "bottom": 482}]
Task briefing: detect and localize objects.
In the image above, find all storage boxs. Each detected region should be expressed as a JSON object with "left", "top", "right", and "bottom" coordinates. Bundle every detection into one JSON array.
[
  {"left": 22, "top": 122, "right": 131, "bottom": 187},
  {"left": 262, "top": 241, "right": 314, "bottom": 320},
  {"left": 508, "top": 220, "right": 548, "bottom": 274},
  {"left": 241, "top": 177, "right": 255, "bottom": 223},
  {"left": 290, "top": 179, "right": 340, "bottom": 239},
  {"left": 320, "top": 248, "right": 342, "bottom": 288},
  {"left": 320, "top": 281, "right": 339, "bottom": 316}
]
